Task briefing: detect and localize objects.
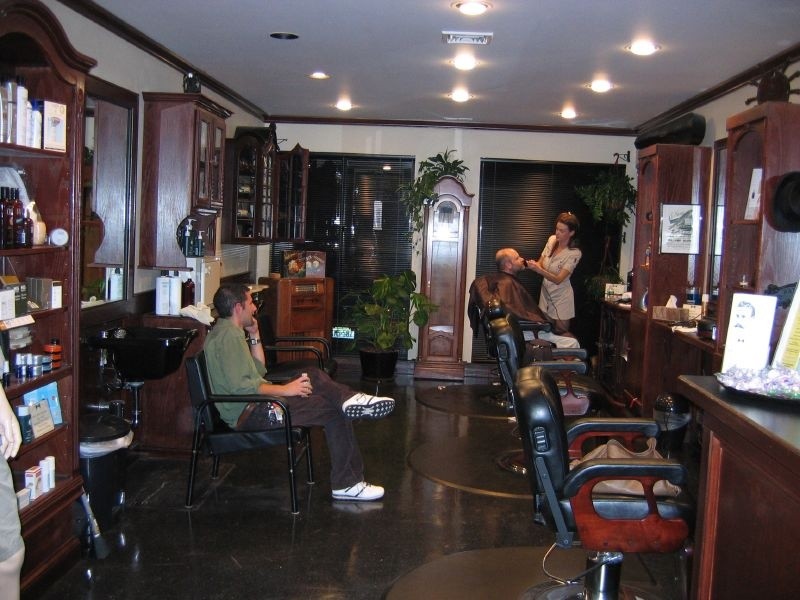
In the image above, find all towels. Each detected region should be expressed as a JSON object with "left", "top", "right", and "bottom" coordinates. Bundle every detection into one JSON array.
[{"left": 181, "top": 302, "right": 215, "bottom": 326}]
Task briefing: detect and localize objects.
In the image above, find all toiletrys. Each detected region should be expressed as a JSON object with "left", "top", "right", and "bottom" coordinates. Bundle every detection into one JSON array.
[
  {"left": 0, "top": 185, "right": 34, "bottom": 249},
  {"left": 17, "top": 405, "right": 32, "bottom": 444},
  {"left": 110, "top": 267, "right": 123, "bottom": 301},
  {"left": 40, "top": 455, "right": 56, "bottom": 493},
  {"left": 0, "top": 80, "right": 45, "bottom": 149},
  {"left": 155, "top": 269, "right": 181, "bottom": 316}
]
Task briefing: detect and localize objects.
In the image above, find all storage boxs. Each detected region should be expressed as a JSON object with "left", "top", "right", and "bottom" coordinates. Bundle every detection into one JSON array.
[
  {"left": 16, "top": 488, "right": 30, "bottom": 511},
  {"left": 25, "top": 466, "right": 42, "bottom": 501}
]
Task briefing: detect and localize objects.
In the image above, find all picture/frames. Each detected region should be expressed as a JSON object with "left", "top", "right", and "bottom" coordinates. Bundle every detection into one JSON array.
[{"left": 659, "top": 202, "right": 702, "bottom": 254}]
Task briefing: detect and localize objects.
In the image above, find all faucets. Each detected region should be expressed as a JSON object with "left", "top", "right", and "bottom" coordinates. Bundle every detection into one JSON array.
[{"left": 99, "top": 327, "right": 120, "bottom": 339}]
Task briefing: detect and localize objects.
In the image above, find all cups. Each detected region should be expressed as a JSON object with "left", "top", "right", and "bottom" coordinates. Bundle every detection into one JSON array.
[
  {"left": 270, "top": 273, "right": 280, "bottom": 278},
  {"left": 622, "top": 292, "right": 631, "bottom": 302}
]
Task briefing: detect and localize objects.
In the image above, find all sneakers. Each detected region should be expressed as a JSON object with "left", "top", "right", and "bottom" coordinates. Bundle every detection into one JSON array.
[
  {"left": 341, "top": 393, "right": 395, "bottom": 418},
  {"left": 332, "top": 479, "right": 384, "bottom": 501}
]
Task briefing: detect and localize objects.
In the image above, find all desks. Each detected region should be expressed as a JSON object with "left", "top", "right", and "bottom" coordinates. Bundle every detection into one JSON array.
[{"left": 678, "top": 374, "right": 800, "bottom": 600}]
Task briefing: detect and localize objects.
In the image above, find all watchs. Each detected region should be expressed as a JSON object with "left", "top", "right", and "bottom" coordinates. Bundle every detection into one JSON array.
[{"left": 248, "top": 338, "right": 261, "bottom": 345}]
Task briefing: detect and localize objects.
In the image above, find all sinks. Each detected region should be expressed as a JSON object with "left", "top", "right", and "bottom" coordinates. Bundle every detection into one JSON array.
[{"left": 89, "top": 326, "right": 199, "bottom": 383}]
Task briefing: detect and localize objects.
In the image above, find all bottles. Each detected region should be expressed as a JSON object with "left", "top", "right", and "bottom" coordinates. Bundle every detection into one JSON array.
[
  {"left": 300, "top": 373, "right": 310, "bottom": 398},
  {"left": 645, "top": 240, "right": 651, "bottom": 265},
  {"left": 0, "top": 81, "right": 42, "bottom": 149},
  {"left": 607, "top": 286, "right": 614, "bottom": 299},
  {"left": 3, "top": 361, "right": 10, "bottom": 387},
  {"left": 15, "top": 354, "right": 33, "bottom": 379},
  {"left": 182, "top": 278, "right": 195, "bottom": 309},
  {"left": 156, "top": 270, "right": 182, "bottom": 316},
  {"left": 627, "top": 269, "right": 633, "bottom": 292},
  {"left": 0, "top": 187, "right": 34, "bottom": 249},
  {"left": 18, "top": 407, "right": 33, "bottom": 444},
  {"left": 110, "top": 267, "right": 123, "bottom": 301}
]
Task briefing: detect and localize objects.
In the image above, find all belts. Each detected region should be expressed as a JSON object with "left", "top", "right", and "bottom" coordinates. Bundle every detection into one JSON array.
[{"left": 236, "top": 402, "right": 257, "bottom": 433}]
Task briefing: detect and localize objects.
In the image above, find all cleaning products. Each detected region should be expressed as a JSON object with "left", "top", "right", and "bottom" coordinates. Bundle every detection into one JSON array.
[{"left": 183, "top": 218, "right": 206, "bottom": 257}]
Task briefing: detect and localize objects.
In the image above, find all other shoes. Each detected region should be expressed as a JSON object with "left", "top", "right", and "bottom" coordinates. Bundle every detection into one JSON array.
[{"left": 589, "top": 397, "right": 601, "bottom": 411}]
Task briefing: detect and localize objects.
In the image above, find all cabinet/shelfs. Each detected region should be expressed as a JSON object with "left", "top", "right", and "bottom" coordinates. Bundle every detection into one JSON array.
[
  {"left": 627, "top": 144, "right": 713, "bottom": 421},
  {"left": 713, "top": 103, "right": 800, "bottom": 374},
  {"left": 0, "top": 0, "right": 333, "bottom": 600},
  {"left": 596, "top": 295, "right": 630, "bottom": 410}
]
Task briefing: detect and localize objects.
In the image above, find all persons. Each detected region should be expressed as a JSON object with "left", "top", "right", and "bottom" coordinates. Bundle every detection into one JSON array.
[
  {"left": 525, "top": 212, "right": 582, "bottom": 335},
  {"left": 203, "top": 282, "right": 395, "bottom": 501},
  {"left": 493, "top": 247, "right": 580, "bottom": 350}
]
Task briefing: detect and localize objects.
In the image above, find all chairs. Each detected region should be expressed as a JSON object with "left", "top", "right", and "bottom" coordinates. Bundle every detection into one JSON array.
[
  {"left": 185, "top": 350, "right": 314, "bottom": 515},
  {"left": 254, "top": 309, "right": 338, "bottom": 446},
  {"left": 472, "top": 275, "right": 695, "bottom": 600}
]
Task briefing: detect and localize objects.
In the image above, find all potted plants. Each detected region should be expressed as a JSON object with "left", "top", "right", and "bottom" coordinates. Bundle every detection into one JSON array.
[{"left": 343, "top": 268, "right": 439, "bottom": 379}]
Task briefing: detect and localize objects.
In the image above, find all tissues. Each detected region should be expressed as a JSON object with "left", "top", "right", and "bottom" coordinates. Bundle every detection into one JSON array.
[{"left": 652, "top": 295, "right": 690, "bottom": 321}]
